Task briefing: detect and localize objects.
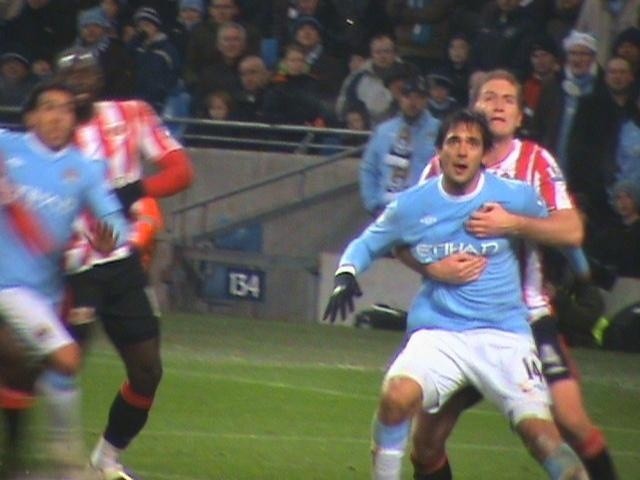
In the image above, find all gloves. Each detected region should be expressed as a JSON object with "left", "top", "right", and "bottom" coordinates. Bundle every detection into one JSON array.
[{"left": 322, "top": 273, "right": 364, "bottom": 323}]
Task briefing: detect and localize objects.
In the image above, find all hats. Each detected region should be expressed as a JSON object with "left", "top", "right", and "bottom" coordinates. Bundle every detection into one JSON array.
[
  {"left": 77, "top": 9, "right": 111, "bottom": 30},
  {"left": 0, "top": 41, "right": 30, "bottom": 66},
  {"left": 395, "top": 74, "right": 426, "bottom": 97},
  {"left": 561, "top": 33, "right": 597, "bottom": 54},
  {"left": 294, "top": 16, "right": 324, "bottom": 37},
  {"left": 132, "top": 6, "right": 165, "bottom": 29},
  {"left": 178, "top": 1, "right": 202, "bottom": 13}
]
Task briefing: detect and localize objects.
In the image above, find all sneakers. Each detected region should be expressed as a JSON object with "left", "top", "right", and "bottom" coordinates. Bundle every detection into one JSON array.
[
  {"left": 592, "top": 316, "right": 609, "bottom": 344},
  {"left": 86, "top": 457, "right": 128, "bottom": 480}
]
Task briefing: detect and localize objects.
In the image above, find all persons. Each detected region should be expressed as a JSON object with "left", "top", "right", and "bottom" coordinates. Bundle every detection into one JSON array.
[
  {"left": 538, "top": 31, "right": 606, "bottom": 309},
  {"left": 2, "top": 80, "right": 128, "bottom": 480},
  {"left": 592, "top": 179, "right": 639, "bottom": 347},
  {"left": 612, "top": 27, "right": 638, "bottom": 63},
  {"left": 0, "top": 2, "right": 405, "bottom": 152},
  {"left": 359, "top": 72, "right": 443, "bottom": 217},
  {"left": 391, "top": 69, "right": 619, "bottom": 480},
  {"left": 3, "top": 43, "right": 198, "bottom": 480},
  {"left": 575, "top": 0, "right": 640, "bottom": 69},
  {"left": 322, "top": 109, "right": 600, "bottom": 480},
  {"left": 567, "top": 56, "right": 640, "bottom": 180},
  {"left": 404, "top": 0, "right": 560, "bottom": 121}
]
